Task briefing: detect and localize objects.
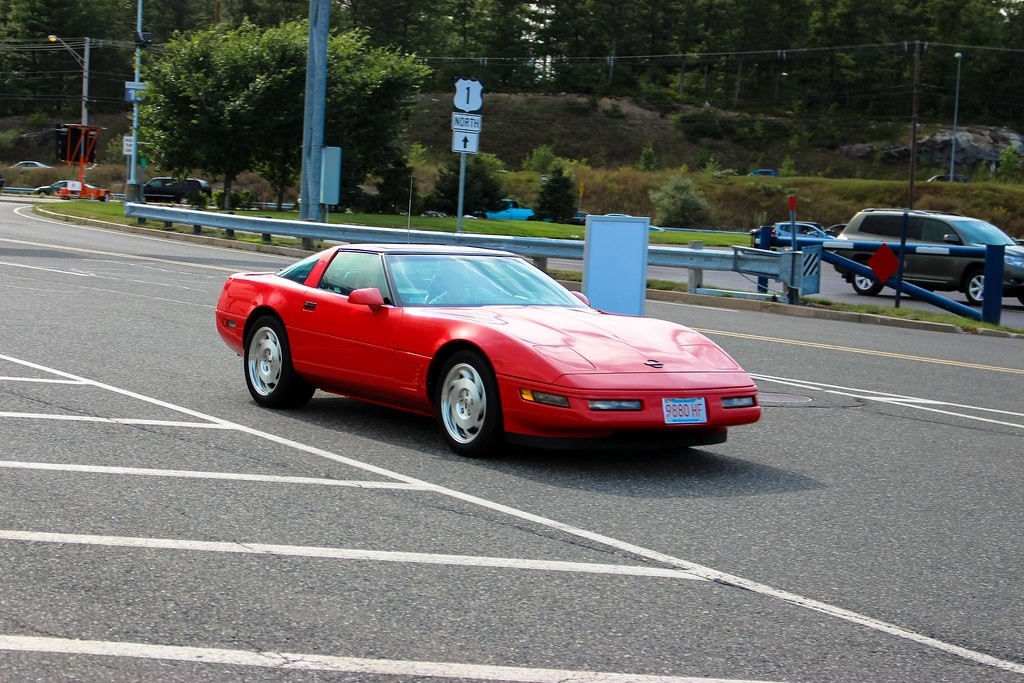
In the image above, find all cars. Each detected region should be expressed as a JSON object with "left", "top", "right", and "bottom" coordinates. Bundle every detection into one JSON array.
[
  {"left": 772, "top": 223, "right": 836, "bottom": 250},
  {"left": 604, "top": 212, "right": 665, "bottom": 231},
  {"left": 808, "top": 224, "right": 848, "bottom": 238},
  {"left": 474, "top": 198, "right": 535, "bottom": 220},
  {"left": 9, "top": 161, "right": 53, "bottom": 168},
  {"left": 36, "top": 180, "right": 73, "bottom": 197},
  {"left": 572, "top": 209, "right": 592, "bottom": 225}
]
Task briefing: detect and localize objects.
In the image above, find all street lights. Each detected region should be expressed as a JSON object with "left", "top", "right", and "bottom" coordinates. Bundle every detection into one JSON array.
[
  {"left": 48, "top": 35, "right": 89, "bottom": 125},
  {"left": 951, "top": 52, "right": 962, "bottom": 181}
]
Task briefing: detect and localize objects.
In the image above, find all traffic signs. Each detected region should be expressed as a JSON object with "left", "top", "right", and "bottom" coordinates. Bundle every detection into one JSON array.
[{"left": 451, "top": 112, "right": 482, "bottom": 155}]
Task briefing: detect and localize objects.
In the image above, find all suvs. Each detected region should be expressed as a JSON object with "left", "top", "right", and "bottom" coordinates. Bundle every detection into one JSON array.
[{"left": 834, "top": 207, "right": 1024, "bottom": 306}]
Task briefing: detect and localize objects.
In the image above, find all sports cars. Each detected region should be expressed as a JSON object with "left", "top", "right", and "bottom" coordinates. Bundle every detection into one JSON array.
[
  {"left": 218, "top": 246, "right": 759, "bottom": 458},
  {"left": 57, "top": 182, "right": 113, "bottom": 202}
]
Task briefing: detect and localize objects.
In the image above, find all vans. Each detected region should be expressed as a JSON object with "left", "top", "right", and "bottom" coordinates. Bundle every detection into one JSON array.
[
  {"left": 926, "top": 174, "right": 969, "bottom": 184},
  {"left": 747, "top": 169, "right": 777, "bottom": 177},
  {"left": 143, "top": 177, "right": 213, "bottom": 205}
]
